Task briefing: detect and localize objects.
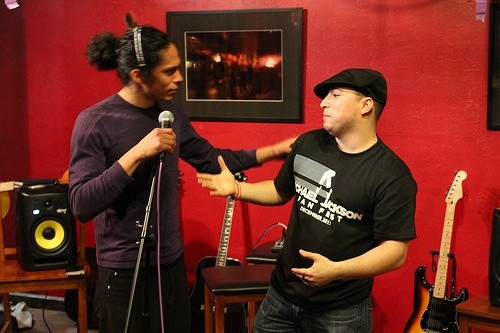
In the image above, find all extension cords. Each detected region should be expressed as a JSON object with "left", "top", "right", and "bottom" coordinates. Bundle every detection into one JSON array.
[{"left": 271, "top": 237, "right": 285, "bottom": 251}]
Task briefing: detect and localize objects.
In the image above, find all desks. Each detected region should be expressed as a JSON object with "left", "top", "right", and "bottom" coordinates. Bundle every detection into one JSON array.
[{"left": 456, "top": 292, "right": 500, "bottom": 333}]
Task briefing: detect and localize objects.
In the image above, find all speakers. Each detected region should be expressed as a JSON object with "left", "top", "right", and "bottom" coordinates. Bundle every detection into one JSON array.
[{"left": 16, "top": 181, "right": 76, "bottom": 271}]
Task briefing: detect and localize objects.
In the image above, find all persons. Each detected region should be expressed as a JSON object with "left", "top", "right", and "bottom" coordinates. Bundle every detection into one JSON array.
[
  {"left": 69, "top": 25, "right": 298, "bottom": 333},
  {"left": 196, "top": 68, "right": 418, "bottom": 333}
]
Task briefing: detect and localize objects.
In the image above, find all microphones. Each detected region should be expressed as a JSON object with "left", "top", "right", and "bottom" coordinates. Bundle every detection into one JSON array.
[{"left": 158, "top": 111, "right": 174, "bottom": 161}]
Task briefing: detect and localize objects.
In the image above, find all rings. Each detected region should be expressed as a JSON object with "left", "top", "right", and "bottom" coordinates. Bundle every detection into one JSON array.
[{"left": 302, "top": 275, "right": 305, "bottom": 281}]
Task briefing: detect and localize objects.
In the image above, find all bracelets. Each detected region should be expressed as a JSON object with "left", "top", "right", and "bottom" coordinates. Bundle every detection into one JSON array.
[{"left": 231, "top": 180, "right": 241, "bottom": 200}]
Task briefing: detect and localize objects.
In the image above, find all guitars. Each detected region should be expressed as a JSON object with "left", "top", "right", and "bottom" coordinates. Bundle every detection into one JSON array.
[
  {"left": 403, "top": 169, "right": 469, "bottom": 333},
  {"left": 187, "top": 170, "right": 248, "bottom": 333}
]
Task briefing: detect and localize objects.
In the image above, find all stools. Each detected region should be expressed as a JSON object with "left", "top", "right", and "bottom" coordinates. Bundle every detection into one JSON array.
[{"left": 201, "top": 264, "right": 275, "bottom": 333}]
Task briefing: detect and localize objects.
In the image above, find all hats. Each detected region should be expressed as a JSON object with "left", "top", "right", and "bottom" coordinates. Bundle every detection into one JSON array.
[{"left": 314, "top": 68, "right": 387, "bottom": 108}]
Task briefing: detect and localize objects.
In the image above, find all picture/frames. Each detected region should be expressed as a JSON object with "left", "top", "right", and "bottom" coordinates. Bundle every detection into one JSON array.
[
  {"left": 166, "top": 7, "right": 302, "bottom": 123},
  {"left": 487, "top": 1, "right": 500, "bottom": 131}
]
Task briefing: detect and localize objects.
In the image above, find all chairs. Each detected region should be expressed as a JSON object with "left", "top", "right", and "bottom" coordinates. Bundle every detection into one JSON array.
[{"left": 0, "top": 192, "right": 91, "bottom": 333}]
